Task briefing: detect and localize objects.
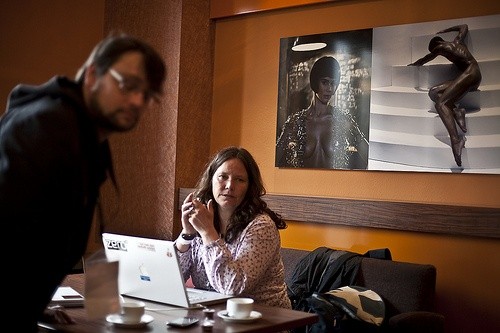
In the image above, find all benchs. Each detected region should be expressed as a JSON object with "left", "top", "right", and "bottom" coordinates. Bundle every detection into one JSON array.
[{"left": 279, "top": 247, "right": 445, "bottom": 333}]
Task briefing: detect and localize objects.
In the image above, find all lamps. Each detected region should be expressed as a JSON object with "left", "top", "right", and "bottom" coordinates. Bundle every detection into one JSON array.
[{"left": 291, "top": 34, "right": 328, "bottom": 52}]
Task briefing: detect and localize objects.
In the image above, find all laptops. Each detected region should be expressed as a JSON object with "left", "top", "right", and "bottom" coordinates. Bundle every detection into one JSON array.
[{"left": 102, "top": 233, "right": 235, "bottom": 310}]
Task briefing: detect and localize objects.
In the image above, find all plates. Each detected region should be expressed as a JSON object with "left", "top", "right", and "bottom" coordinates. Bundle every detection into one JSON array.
[
  {"left": 105, "top": 313, "right": 153, "bottom": 327},
  {"left": 217, "top": 309, "right": 262, "bottom": 322}
]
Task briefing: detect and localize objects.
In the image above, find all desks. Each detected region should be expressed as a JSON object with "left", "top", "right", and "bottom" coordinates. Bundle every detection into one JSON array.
[{"left": 34, "top": 274, "right": 319, "bottom": 333}]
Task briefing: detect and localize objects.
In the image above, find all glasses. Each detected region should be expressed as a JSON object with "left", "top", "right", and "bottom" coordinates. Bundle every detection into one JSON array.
[{"left": 107, "top": 67, "right": 162, "bottom": 109}]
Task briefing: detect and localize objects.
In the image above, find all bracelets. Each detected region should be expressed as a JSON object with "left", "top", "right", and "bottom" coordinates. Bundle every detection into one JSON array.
[{"left": 181, "top": 232, "right": 196, "bottom": 240}]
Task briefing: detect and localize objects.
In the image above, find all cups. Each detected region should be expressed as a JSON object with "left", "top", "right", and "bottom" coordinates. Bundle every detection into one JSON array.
[
  {"left": 227, "top": 298, "right": 254, "bottom": 318},
  {"left": 118, "top": 303, "right": 145, "bottom": 323}
]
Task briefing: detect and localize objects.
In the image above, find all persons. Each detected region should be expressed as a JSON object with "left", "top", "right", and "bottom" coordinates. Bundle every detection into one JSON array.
[
  {"left": 277, "top": 56, "right": 370, "bottom": 170},
  {"left": 1, "top": 33, "right": 167, "bottom": 333},
  {"left": 173, "top": 147, "right": 292, "bottom": 311}
]
